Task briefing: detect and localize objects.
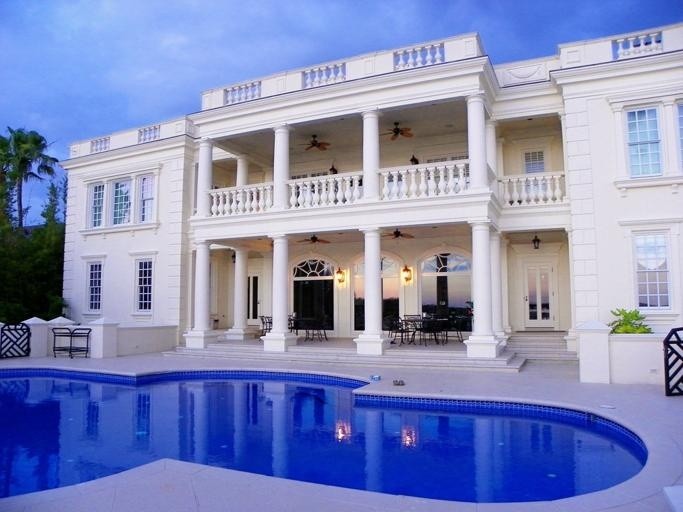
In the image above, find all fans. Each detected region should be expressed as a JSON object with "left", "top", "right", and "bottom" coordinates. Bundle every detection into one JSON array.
[
  {"left": 385, "top": 121, "right": 414, "bottom": 141},
  {"left": 382, "top": 229, "right": 416, "bottom": 240},
  {"left": 296, "top": 234, "right": 331, "bottom": 245},
  {"left": 298, "top": 135, "right": 331, "bottom": 151}
]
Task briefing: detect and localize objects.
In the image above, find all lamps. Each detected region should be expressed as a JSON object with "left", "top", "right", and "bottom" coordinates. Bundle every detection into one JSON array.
[
  {"left": 232, "top": 252, "right": 235, "bottom": 263},
  {"left": 329, "top": 158, "right": 337, "bottom": 174},
  {"left": 338, "top": 426, "right": 347, "bottom": 443},
  {"left": 406, "top": 431, "right": 413, "bottom": 447},
  {"left": 409, "top": 154, "right": 419, "bottom": 165},
  {"left": 402, "top": 265, "right": 411, "bottom": 281},
  {"left": 336, "top": 267, "right": 345, "bottom": 283},
  {"left": 532, "top": 236, "right": 541, "bottom": 249}
]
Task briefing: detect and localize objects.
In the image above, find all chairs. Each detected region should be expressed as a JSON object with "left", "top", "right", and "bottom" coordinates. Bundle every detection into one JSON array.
[
  {"left": 388, "top": 314, "right": 463, "bottom": 347},
  {"left": 257, "top": 314, "right": 329, "bottom": 342}
]
